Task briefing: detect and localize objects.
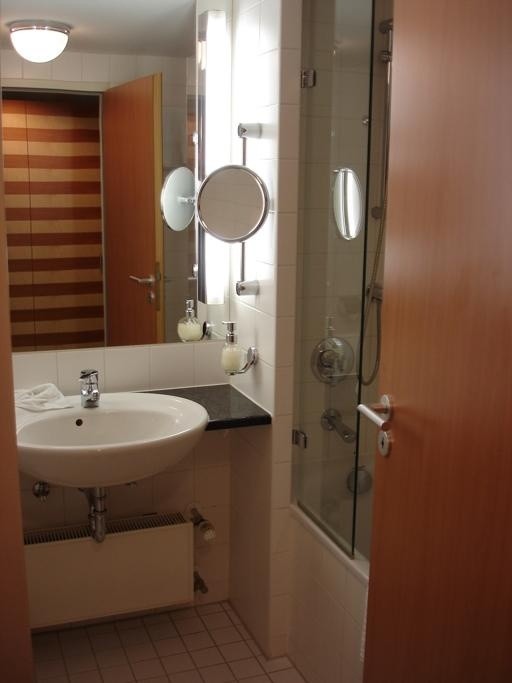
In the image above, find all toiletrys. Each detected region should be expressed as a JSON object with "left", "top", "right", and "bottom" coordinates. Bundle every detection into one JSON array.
[
  {"left": 175, "top": 297, "right": 203, "bottom": 342},
  {"left": 222, "top": 321, "right": 242, "bottom": 373}
]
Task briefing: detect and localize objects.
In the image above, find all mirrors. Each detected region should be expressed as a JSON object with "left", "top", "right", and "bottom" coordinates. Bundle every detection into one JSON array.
[
  {"left": 192, "top": 166, "right": 269, "bottom": 242},
  {"left": 5, "top": 19, "right": 73, "bottom": 65},
  {"left": 159, "top": 168, "right": 195, "bottom": 232},
  {"left": 330, "top": 168, "right": 364, "bottom": 242},
  {"left": 0, "top": 0, "right": 233, "bottom": 353}
]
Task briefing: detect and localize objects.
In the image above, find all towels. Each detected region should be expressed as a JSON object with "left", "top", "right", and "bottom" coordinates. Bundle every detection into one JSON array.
[{"left": 14, "top": 382, "right": 74, "bottom": 411}]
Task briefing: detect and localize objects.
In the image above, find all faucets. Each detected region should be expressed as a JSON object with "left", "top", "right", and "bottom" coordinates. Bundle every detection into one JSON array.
[{"left": 78, "top": 368, "right": 100, "bottom": 408}]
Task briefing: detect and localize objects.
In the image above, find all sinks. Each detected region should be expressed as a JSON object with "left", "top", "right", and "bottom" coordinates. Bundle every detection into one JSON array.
[{"left": 15, "top": 395, "right": 210, "bottom": 488}]
